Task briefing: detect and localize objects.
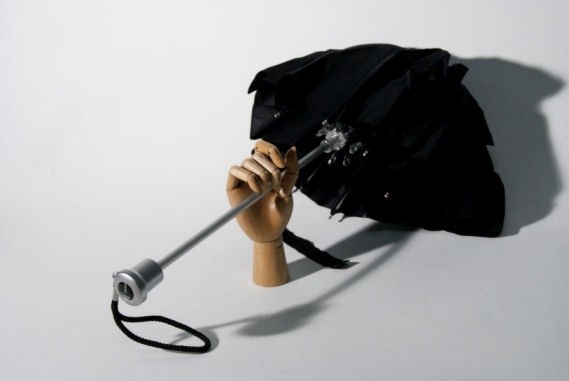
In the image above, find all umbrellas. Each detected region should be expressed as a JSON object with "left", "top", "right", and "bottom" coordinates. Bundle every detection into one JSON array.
[{"left": 111, "top": 44, "right": 504, "bottom": 353}]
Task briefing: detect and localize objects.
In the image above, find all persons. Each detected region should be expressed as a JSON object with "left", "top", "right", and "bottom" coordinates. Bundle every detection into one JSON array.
[{"left": 226, "top": 141, "right": 300, "bottom": 287}]
